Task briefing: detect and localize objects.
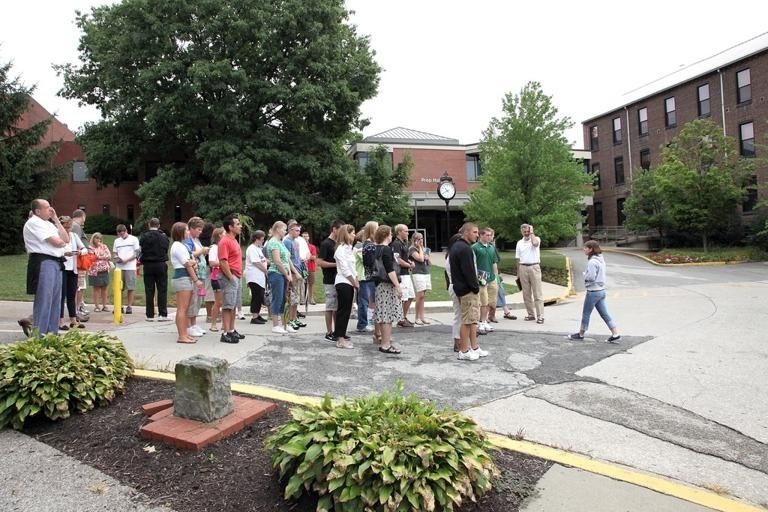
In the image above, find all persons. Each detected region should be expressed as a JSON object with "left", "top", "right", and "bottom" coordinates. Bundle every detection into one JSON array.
[
  {"left": 568, "top": 240, "right": 622, "bottom": 343},
  {"left": 514, "top": 223, "right": 545, "bottom": 323},
  {"left": 445, "top": 222, "right": 516, "bottom": 361},
  {"left": 18, "top": 199, "right": 141, "bottom": 338},
  {"left": 138, "top": 216, "right": 433, "bottom": 354}
]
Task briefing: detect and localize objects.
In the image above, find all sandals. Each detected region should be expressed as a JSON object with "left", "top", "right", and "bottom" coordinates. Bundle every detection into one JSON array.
[{"left": 379, "top": 345, "right": 401, "bottom": 355}]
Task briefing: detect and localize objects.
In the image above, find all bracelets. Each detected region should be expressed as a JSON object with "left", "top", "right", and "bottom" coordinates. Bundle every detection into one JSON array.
[{"left": 530, "top": 233, "right": 534, "bottom": 235}]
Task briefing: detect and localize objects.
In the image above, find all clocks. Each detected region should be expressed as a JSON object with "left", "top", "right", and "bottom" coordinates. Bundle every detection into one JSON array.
[{"left": 437, "top": 180, "right": 456, "bottom": 200}]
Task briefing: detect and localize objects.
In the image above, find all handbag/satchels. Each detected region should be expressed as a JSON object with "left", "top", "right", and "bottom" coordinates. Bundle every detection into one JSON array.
[
  {"left": 76, "top": 246, "right": 97, "bottom": 272},
  {"left": 371, "top": 246, "right": 402, "bottom": 283}
]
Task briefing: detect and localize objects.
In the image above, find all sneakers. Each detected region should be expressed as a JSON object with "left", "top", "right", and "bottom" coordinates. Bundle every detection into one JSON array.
[
  {"left": 59, "top": 302, "right": 90, "bottom": 331},
  {"left": 125, "top": 306, "right": 133, "bottom": 315},
  {"left": 476, "top": 318, "right": 499, "bottom": 335},
  {"left": 252, "top": 316, "right": 307, "bottom": 334},
  {"left": 536, "top": 318, "right": 546, "bottom": 325},
  {"left": 220, "top": 330, "right": 246, "bottom": 344},
  {"left": 157, "top": 315, "right": 173, "bottom": 323},
  {"left": 457, "top": 350, "right": 479, "bottom": 361},
  {"left": 93, "top": 305, "right": 110, "bottom": 314},
  {"left": 177, "top": 325, "right": 207, "bottom": 344},
  {"left": 503, "top": 312, "right": 517, "bottom": 320},
  {"left": 324, "top": 330, "right": 354, "bottom": 349},
  {"left": 604, "top": 334, "right": 621, "bottom": 344},
  {"left": 567, "top": 332, "right": 585, "bottom": 342},
  {"left": 18, "top": 316, "right": 33, "bottom": 338},
  {"left": 355, "top": 325, "right": 374, "bottom": 335},
  {"left": 469, "top": 345, "right": 489, "bottom": 358},
  {"left": 523, "top": 316, "right": 536, "bottom": 322},
  {"left": 144, "top": 316, "right": 154, "bottom": 323},
  {"left": 397, "top": 317, "right": 431, "bottom": 330}
]
